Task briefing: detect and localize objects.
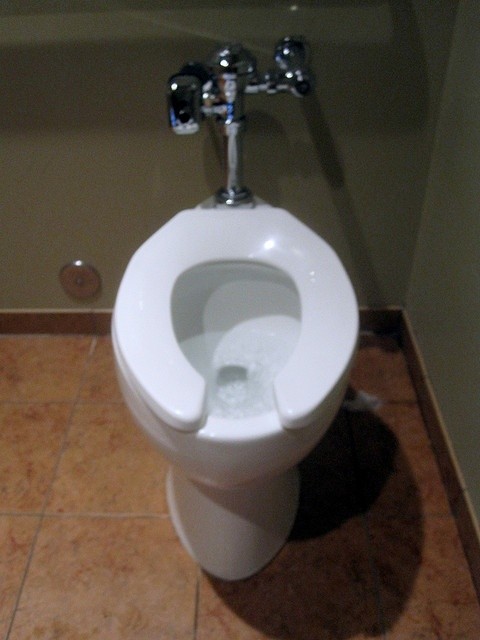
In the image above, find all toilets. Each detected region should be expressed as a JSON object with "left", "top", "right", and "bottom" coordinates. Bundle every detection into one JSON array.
[{"left": 110, "top": 37, "right": 360, "bottom": 581}]
